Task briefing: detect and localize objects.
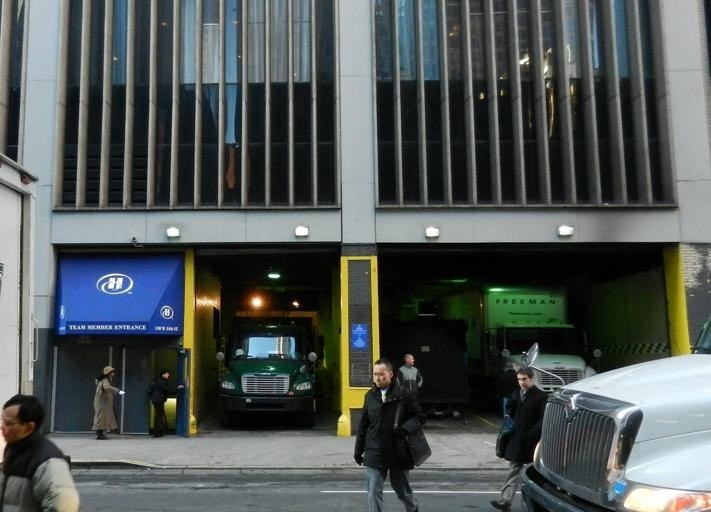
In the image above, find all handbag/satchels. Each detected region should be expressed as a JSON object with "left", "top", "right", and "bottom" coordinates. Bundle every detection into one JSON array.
[
  {"left": 496, "top": 432, "right": 512, "bottom": 457},
  {"left": 395, "top": 416, "right": 431, "bottom": 466}
]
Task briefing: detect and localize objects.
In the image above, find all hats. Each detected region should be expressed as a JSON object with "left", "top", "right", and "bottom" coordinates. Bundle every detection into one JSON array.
[{"left": 104, "top": 366, "right": 115, "bottom": 375}]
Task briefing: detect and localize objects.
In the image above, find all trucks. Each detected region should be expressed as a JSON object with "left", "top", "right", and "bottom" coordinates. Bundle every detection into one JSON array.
[
  {"left": 516, "top": 307, "right": 711, "bottom": 511},
  {"left": 216, "top": 311, "right": 327, "bottom": 427},
  {"left": 426, "top": 285, "right": 596, "bottom": 407}
]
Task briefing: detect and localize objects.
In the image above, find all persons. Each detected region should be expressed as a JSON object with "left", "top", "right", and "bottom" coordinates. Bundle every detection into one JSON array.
[
  {"left": 354, "top": 357, "right": 428, "bottom": 511},
  {"left": 0, "top": 393, "right": 81, "bottom": 512},
  {"left": 151, "top": 368, "right": 185, "bottom": 438},
  {"left": 490, "top": 367, "right": 548, "bottom": 511},
  {"left": 91, "top": 365, "right": 126, "bottom": 439},
  {"left": 499, "top": 361, "right": 518, "bottom": 420},
  {"left": 396, "top": 353, "right": 424, "bottom": 393}
]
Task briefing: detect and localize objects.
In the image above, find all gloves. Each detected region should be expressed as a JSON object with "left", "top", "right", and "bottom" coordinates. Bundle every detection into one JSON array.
[
  {"left": 119, "top": 391, "right": 125, "bottom": 395},
  {"left": 355, "top": 454, "right": 364, "bottom": 465},
  {"left": 397, "top": 428, "right": 408, "bottom": 440}
]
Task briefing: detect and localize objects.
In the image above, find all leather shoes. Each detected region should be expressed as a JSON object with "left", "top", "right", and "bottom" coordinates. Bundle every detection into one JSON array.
[{"left": 491, "top": 500, "right": 511, "bottom": 511}]
